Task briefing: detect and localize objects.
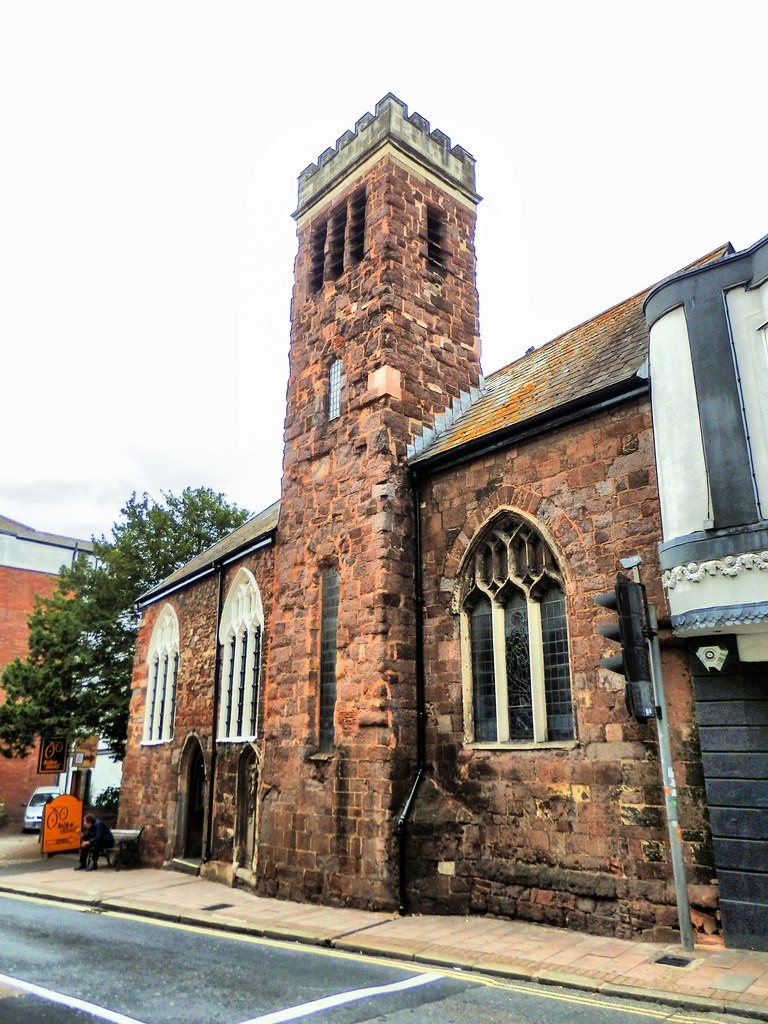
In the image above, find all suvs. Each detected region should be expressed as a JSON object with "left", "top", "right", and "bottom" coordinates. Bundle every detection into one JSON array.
[{"left": 21, "top": 786, "right": 63, "bottom": 833}]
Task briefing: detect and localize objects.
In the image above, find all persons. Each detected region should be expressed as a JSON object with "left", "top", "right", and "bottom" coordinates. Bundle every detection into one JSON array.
[{"left": 74, "top": 814, "right": 114, "bottom": 871}]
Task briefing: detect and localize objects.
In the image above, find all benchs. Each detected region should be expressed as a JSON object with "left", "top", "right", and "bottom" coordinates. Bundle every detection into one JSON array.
[{"left": 79, "top": 826, "right": 144, "bottom": 872}]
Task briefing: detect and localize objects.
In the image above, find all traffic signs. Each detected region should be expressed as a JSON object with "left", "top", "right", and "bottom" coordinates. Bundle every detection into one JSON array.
[{"left": 72, "top": 735, "right": 99, "bottom": 769}]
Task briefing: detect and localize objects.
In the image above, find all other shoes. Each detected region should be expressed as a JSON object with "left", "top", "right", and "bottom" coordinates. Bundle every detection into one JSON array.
[
  {"left": 74, "top": 864, "right": 86, "bottom": 870},
  {"left": 86, "top": 863, "right": 98, "bottom": 871}
]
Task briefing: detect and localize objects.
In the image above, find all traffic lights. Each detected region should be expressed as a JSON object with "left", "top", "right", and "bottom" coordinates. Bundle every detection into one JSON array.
[
  {"left": 625, "top": 681, "right": 657, "bottom": 725},
  {"left": 594, "top": 573, "right": 653, "bottom": 681}
]
